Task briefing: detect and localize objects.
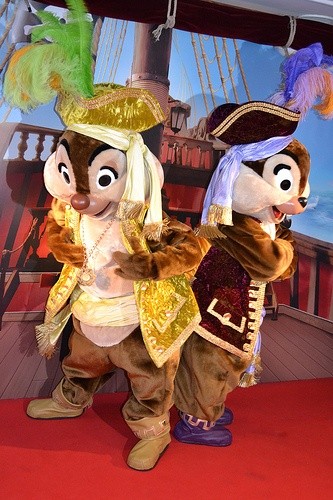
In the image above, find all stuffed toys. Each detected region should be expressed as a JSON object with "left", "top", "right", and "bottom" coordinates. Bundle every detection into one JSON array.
[
  {"left": 172, "top": 99, "right": 311, "bottom": 446},
  {"left": 26, "top": 81, "right": 204, "bottom": 471}
]
